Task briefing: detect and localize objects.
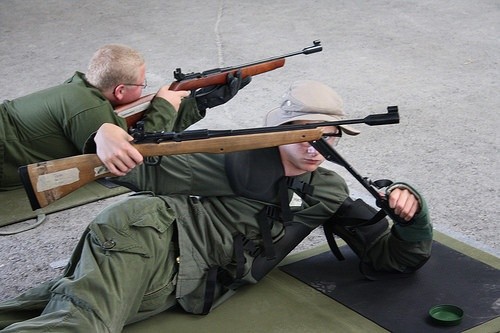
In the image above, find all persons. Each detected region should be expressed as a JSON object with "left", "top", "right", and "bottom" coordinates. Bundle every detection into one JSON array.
[
  {"left": 0, "top": 44, "right": 251, "bottom": 189},
  {"left": 0, "top": 81, "right": 433, "bottom": 333}
]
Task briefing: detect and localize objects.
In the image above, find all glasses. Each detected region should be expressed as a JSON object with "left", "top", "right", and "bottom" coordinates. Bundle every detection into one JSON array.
[
  {"left": 112, "top": 77, "right": 147, "bottom": 93},
  {"left": 321, "top": 126, "right": 342, "bottom": 146}
]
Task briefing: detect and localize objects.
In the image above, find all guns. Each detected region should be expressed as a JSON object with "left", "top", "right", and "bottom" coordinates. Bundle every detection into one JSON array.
[
  {"left": 111, "top": 38, "right": 323, "bottom": 133},
  {"left": 15, "top": 105, "right": 412, "bottom": 229}
]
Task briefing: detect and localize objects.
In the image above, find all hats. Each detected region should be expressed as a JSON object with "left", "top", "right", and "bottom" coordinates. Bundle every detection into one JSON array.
[{"left": 265, "top": 79, "right": 360, "bottom": 135}]
